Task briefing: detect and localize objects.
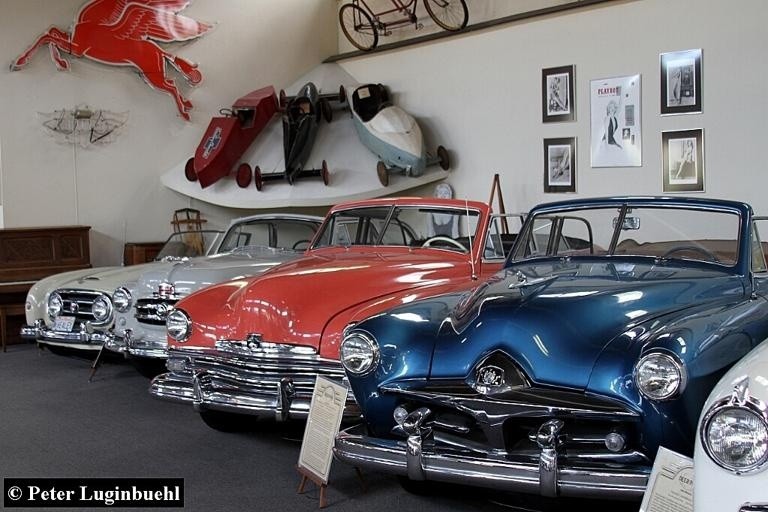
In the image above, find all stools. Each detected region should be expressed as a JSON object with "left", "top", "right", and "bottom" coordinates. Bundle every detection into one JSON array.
[{"left": 0, "top": 301, "right": 25, "bottom": 354}]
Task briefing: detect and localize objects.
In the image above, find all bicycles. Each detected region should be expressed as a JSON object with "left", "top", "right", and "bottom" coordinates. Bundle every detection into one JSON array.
[{"left": 337, "top": 0, "right": 469, "bottom": 52}]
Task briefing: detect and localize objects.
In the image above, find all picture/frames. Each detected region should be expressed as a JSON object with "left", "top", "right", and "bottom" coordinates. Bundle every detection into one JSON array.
[
  {"left": 542, "top": 135, "right": 576, "bottom": 194},
  {"left": 660, "top": 126, "right": 706, "bottom": 196},
  {"left": 541, "top": 64, "right": 577, "bottom": 125},
  {"left": 656, "top": 47, "right": 704, "bottom": 118}
]
